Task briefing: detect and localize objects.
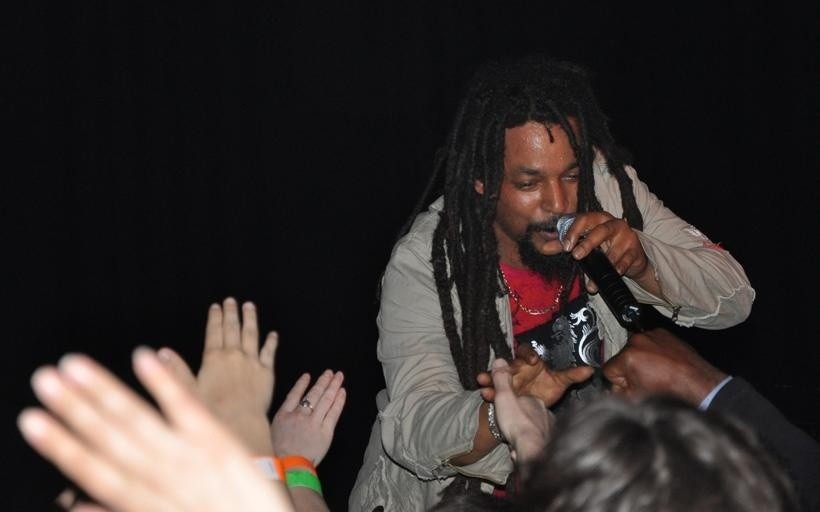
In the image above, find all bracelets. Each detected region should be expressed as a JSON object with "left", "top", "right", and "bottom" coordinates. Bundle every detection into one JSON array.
[
  {"left": 249, "top": 455, "right": 287, "bottom": 481},
  {"left": 278, "top": 456, "right": 322, "bottom": 495}
]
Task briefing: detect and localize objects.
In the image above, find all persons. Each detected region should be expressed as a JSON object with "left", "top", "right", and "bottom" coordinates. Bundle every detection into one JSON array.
[
  {"left": 491, "top": 358, "right": 802, "bottom": 511},
  {"left": 428, "top": 489, "right": 517, "bottom": 512},
  {"left": 158, "top": 297, "right": 297, "bottom": 510},
  {"left": 18, "top": 346, "right": 293, "bottom": 510},
  {"left": 602, "top": 328, "right": 819, "bottom": 511},
  {"left": 269, "top": 369, "right": 346, "bottom": 511},
  {"left": 349, "top": 61, "right": 757, "bottom": 512}
]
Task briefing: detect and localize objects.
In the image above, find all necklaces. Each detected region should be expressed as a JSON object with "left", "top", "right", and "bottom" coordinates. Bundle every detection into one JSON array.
[{"left": 497, "top": 266, "right": 564, "bottom": 315}]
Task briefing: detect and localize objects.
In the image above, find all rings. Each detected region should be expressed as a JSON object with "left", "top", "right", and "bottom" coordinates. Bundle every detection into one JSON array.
[{"left": 299, "top": 399, "right": 314, "bottom": 410}]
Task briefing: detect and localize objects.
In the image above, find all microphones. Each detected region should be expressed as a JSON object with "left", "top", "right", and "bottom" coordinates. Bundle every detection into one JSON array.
[{"left": 556, "top": 214, "right": 646, "bottom": 333}]
